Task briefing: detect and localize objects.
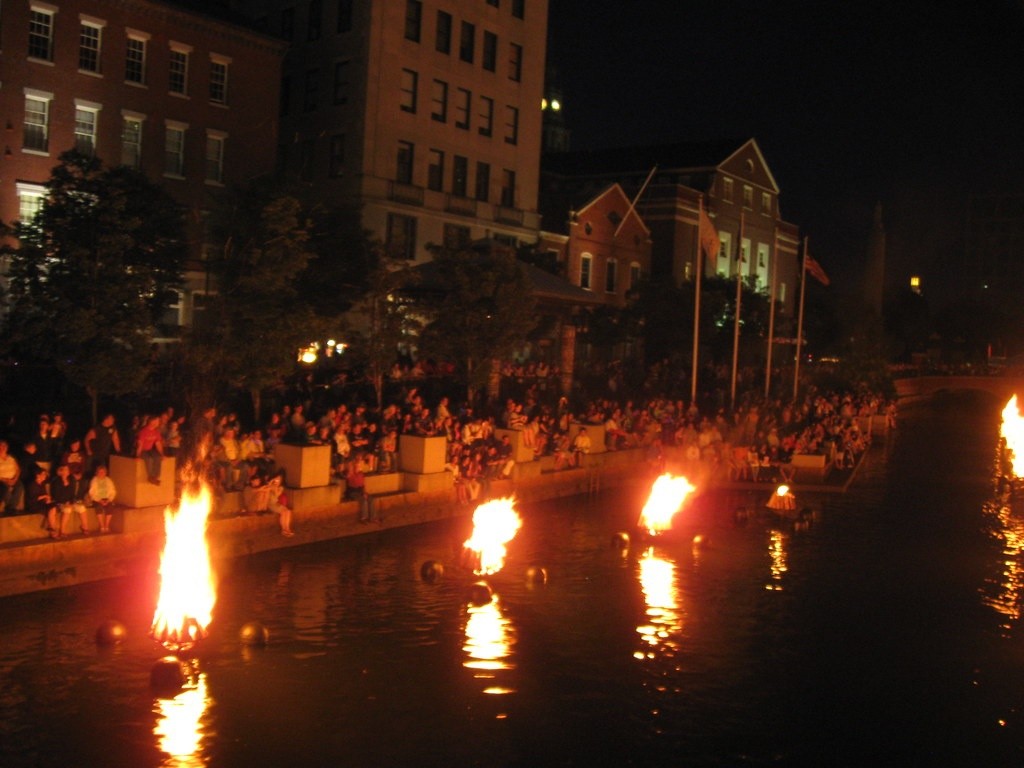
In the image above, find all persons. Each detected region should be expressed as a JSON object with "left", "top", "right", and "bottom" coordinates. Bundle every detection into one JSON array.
[
  {"left": 135, "top": 415, "right": 165, "bottom": 486},
  {"left": 0, "top": 344, "right": 997, "bottom": 541}
]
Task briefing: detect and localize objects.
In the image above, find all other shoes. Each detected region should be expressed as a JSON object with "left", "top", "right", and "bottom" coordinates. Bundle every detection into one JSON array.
[
  {"left": 80, "top": 526, "right": 89, "bottom": 535},
  {"left": 51, "top": 534, "right": 67, "bottom": 540},
  {"left": 282, "top": 530, "right": 295, "bottom": 538},
  {"left": 724, "top": 478, "right": 794, "bottom": 485},
  {"left": 101, "top": 528, "right": 110, "bottom": 534}
]
